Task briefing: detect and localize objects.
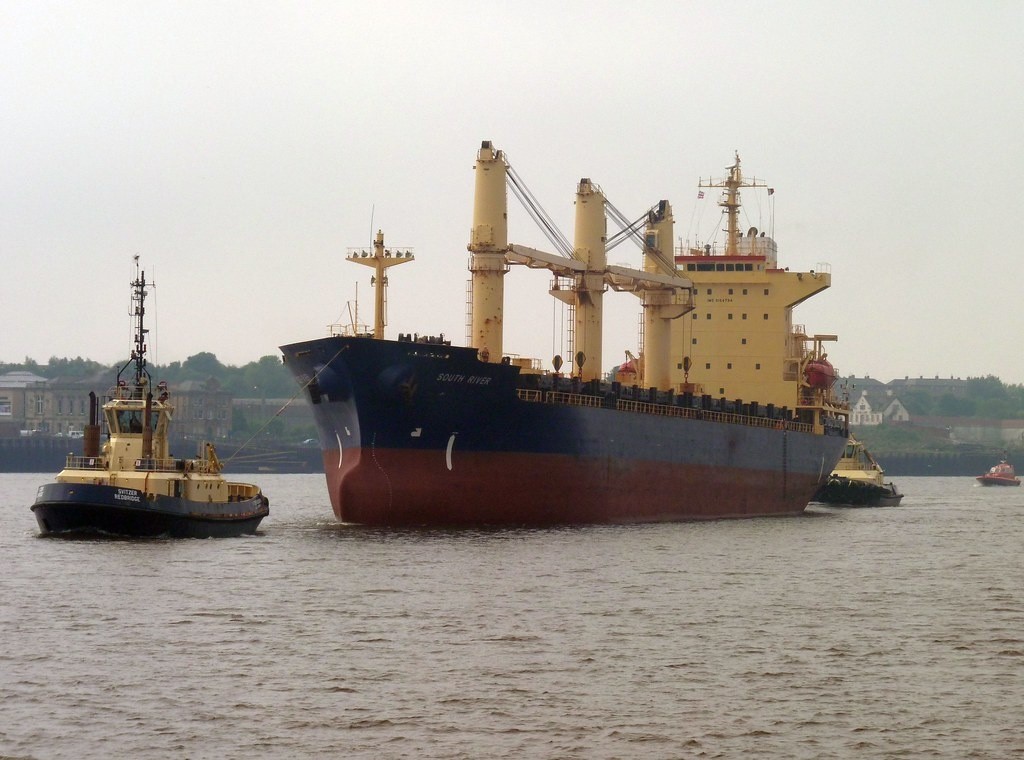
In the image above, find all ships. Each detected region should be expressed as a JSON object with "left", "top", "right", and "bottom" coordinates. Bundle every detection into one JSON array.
[{"left": 275, "top": 141, "right": 856, "bottom": 530}]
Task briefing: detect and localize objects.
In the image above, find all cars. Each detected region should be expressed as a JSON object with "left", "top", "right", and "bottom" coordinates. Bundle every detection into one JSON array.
[{"left": 65, "top": 430, "right": 84, "bottom": 439}]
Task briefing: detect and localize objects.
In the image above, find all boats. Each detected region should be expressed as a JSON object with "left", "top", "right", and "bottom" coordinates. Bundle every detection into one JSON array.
[
  {"left": 976, "top": 460, "right": 1021, "bottom": 486},
  {"left": 811, "top": 431, "right": 904, "bottom": 507},
  {"left": 27, "top": 253, "right": 272, "bottom": 538}
]
